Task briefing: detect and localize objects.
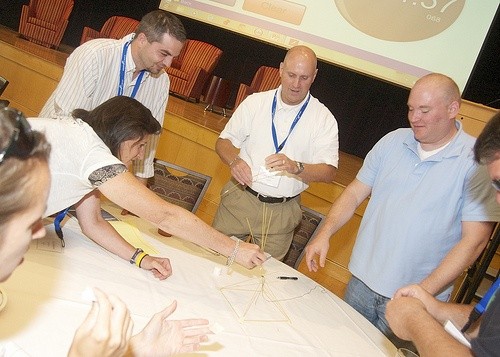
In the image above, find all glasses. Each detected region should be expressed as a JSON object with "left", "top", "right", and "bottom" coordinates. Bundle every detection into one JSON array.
[
  {"left": 491, "top": 179, "right": 500, "bottom": 191},
  {"left": 1, "top": 108, "right": 34, "bottom": 166}
]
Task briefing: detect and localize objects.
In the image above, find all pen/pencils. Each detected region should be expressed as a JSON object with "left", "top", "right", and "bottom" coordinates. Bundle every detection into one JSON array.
[{"left": 278, "top": 277, "right": 298, "bottom": 280}]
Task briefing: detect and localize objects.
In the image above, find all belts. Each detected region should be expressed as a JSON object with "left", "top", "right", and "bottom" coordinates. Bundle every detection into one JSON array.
[{"left": 244, "top": 186, "right": 300, "bottom": 203}]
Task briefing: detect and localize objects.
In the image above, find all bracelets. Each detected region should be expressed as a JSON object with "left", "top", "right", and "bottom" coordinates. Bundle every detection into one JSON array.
[
  {"left": 229, "top": 158, "right": 241, "bottom": 166},
  {"left": 136, "top": 252, "right": 149, "bottom": 268},
  {"left": 225, "top": 240, "right": 241, "bottom": 274}
]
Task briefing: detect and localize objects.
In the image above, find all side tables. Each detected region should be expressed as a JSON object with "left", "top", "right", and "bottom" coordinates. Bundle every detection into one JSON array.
[{"left": 203, "top": 75, "right": 234, "bottom": 117}]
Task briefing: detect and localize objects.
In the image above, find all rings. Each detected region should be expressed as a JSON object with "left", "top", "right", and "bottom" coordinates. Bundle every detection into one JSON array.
[{"left": 283, "top": 160, "right": 285, "bottom": 164}]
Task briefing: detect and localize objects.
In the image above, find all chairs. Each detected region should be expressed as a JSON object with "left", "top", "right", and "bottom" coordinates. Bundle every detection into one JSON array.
[
  {"left": 147, "top": 160, "right": 213, "bottom": 213},
  {"left": 282, "top": 205, "right": 326, "bottom": 269}
]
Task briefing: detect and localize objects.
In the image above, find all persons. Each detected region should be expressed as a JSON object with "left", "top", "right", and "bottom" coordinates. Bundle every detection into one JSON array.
[
  {"left": 385, "top": 111, "right": 500, "bottom": 357},
  {"left": 0, "top": 102, "right": 212, "bottom": 357},
  {"left": 36, "top": 11, "right": 186, "bottom": 215},
  {"left": 306, "top": 73, "right": 500, "bottom": 350},
  {"left": 215, "top": 46, "right": 339, "bottom": 262},
  {"left": 26, "top": 95, "right": 267, "bottom": 279}
]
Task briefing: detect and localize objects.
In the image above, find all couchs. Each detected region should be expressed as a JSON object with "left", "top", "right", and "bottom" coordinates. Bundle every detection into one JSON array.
[
  {"left": 80, "top": 16, "right": 140, "bottom": 45},
  {"left": 16, "top": 0, "right": 74, "bottom": 49},
  {"left": 165, "top": 39, "right": 223, "bottom": 103},
  {"left": 235, "top": 66, "right": 281, "bottom": 109}
]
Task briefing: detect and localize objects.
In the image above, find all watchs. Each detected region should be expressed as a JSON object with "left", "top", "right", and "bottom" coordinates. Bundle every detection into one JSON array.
[
  {"left": 294, "top": 160, "right": 304, "bottom": 176},
  {"left": 129, "top": 248, "right": 144, "bottom": 265}
]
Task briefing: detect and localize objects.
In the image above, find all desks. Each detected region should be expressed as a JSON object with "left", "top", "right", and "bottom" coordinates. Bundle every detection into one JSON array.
[{"left": 0, "top": 220, "right": 404, "bottom": 357}]
